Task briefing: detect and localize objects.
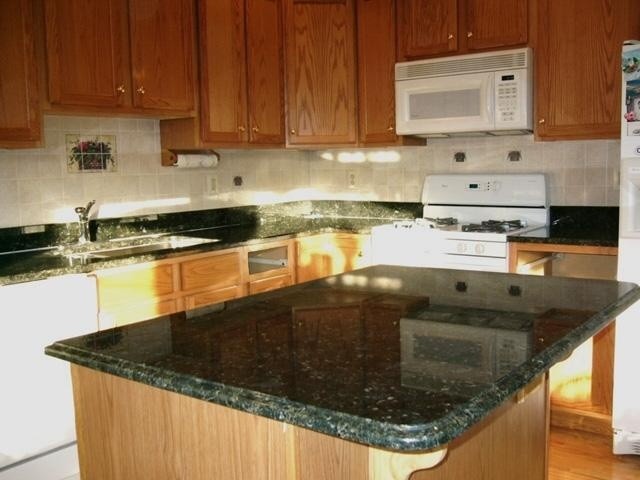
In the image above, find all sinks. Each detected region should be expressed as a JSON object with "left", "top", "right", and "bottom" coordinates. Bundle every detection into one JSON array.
[{"left": 89, "top": 235, "right": 222, "bottom": 259}]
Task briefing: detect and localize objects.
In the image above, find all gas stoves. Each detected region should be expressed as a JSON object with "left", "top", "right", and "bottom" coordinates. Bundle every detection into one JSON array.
[{"left": 372, "top": 217, "right": 550, "bottom": 259}]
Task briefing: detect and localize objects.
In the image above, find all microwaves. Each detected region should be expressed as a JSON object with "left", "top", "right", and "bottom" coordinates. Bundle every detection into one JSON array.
[
  {"left": 395, "top": 46, "right": 535, "bottom": 139},
  {"left": 398, "top": 299, "right": 534, "bottom": 400}
]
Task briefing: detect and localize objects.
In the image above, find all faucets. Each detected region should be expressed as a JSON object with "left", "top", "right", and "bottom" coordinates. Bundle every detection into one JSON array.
[{"left": 76, "top": 200, "right": 96, "bottom": 243}]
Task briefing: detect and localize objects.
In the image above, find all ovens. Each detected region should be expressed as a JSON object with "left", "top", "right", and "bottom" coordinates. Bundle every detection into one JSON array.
[{"left": 373, "top": 248, "right": 545, "bottom": 276}]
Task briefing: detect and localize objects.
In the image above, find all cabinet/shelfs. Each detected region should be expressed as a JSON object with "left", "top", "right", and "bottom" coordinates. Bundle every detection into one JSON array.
[
  {"left": 508, "top": 243, "right": 614, "bottom": 435},
  {"left": 534, "top": 1, "right": 639, "bottom": 141},
  {"left": 98, "top": 249, "right": 249, "bottom": 330},
  {"left": 250, "top": 232, "right": 367, "bottom": 294},
  {"left": 360, "top": 1, "right": 398, "bottom": 146},
  {"left": 198, "top": 0, "right": 285, "bottom": 144},
  {"left": 401, "top": 0, "right": 527, "bottom": 56},
  {"left": 282, "top": 2, "right": 360, "bottom": 149},
  {"left": 0, "top": 1, "right": 42, "bottom": 149},
  {"left": 45, "top": 2, "right": 195, "bottom": 116}
]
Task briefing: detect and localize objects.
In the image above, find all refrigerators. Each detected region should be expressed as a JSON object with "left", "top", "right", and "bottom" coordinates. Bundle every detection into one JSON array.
[{"left": 610, "top": 38, "right": 639, "bottom": 458}]
